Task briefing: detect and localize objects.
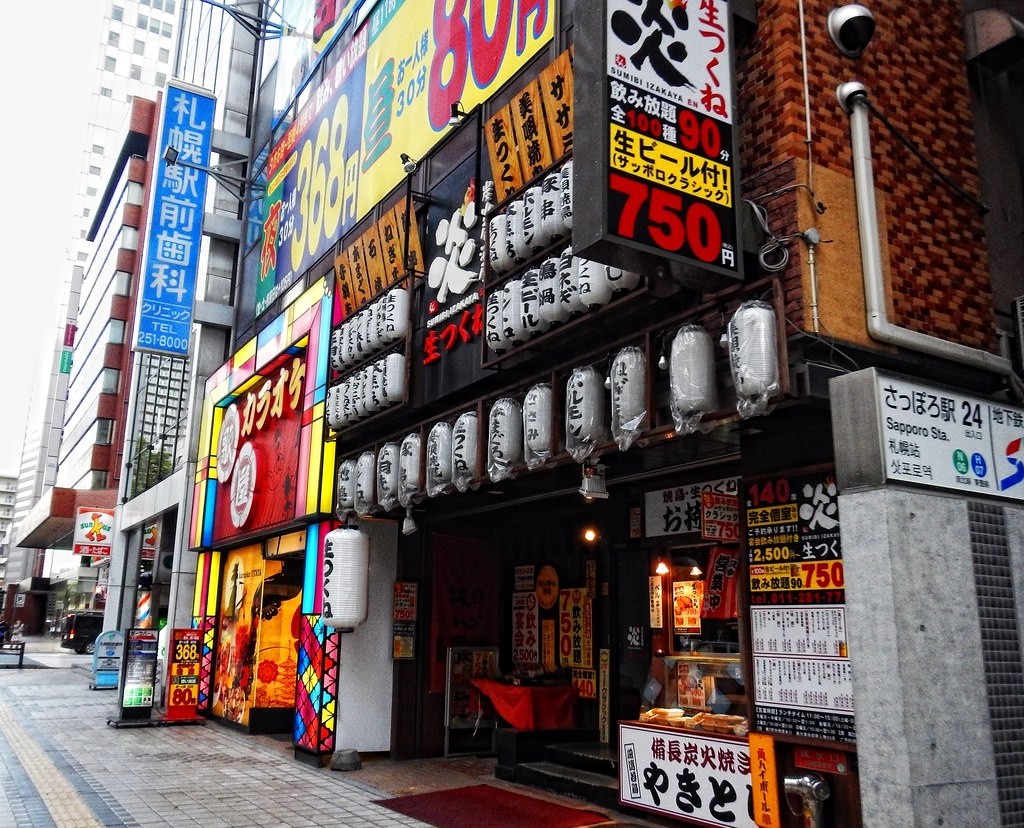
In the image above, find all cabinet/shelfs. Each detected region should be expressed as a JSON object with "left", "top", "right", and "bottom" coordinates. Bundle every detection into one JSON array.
[{"left": 618, "top": 651, "right": 761, "bottom": 828}]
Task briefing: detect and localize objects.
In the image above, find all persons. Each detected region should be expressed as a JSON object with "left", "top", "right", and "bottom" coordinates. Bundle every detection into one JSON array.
[{"left": 0, "top": 612, "right": 25, "bottom": 650}]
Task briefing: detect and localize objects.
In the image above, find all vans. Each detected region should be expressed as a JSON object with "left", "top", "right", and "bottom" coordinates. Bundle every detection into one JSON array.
[{"left": 61, "top": 611, "right": 104, "bottom": 654}]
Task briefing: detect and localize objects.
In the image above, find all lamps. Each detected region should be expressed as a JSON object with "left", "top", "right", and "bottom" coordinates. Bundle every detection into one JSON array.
[
  {"left": 578, "top": 455, "right": 611, "bottom": 502},
  {"left": 402, "top": 505, "right": 419, "bottom": 537},
  {"left": 655, "top": 553, "right": 670, "bottom": 575},
  {"left": 399, "top": 153, "right": 422, "bottom": 174},
  {"left": 448, "top": 101, "right": 471, "bottom": 128},
  {"left": 688, "top": 558, "right": 703, "bottom": 578}
]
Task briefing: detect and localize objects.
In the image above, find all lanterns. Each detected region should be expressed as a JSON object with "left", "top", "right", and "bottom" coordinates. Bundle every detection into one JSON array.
[
  {"left": 337, "top": 458, "right": 356, "bottom": 508},
  {"left": 398, "top": 432, "right": 422, "bottom": 500},
  {"left": 324, "top": 283, "right": 412, "bottom": 433},
  {"left": 354, "top": 450, "right": 376, "bottom": 512},
  {"left": 669, "top": 322, "right": 718, "bottom": 418},
  {"left": 564, "top": 365, "right": 606, "bottom": 450},
  {"left": 322, "top": 524, "right": 369, "bottom": 633},
  {"left": 376, "top": 441, "right": 399, "bottom": 505},
  {"left": 522, "top": 383, "right": 553, "bottom": 464},
  {"left": 486, "top": 157, "right": 646, "bottom": 357},
  {"left": 612, "top": 346, "right": 647, "bottom": 447},
  {"left": 450, "top": 410, "right": 479, "bottom": 480},
  {"left": 728, "top": 300, "right": 782, "bottom": 415},
  {"left": 424, "top": 421, "right": 452, "bottom": 488},
  {"left": 487, "top": 398, "right": 520, "bottom": 468}
]
